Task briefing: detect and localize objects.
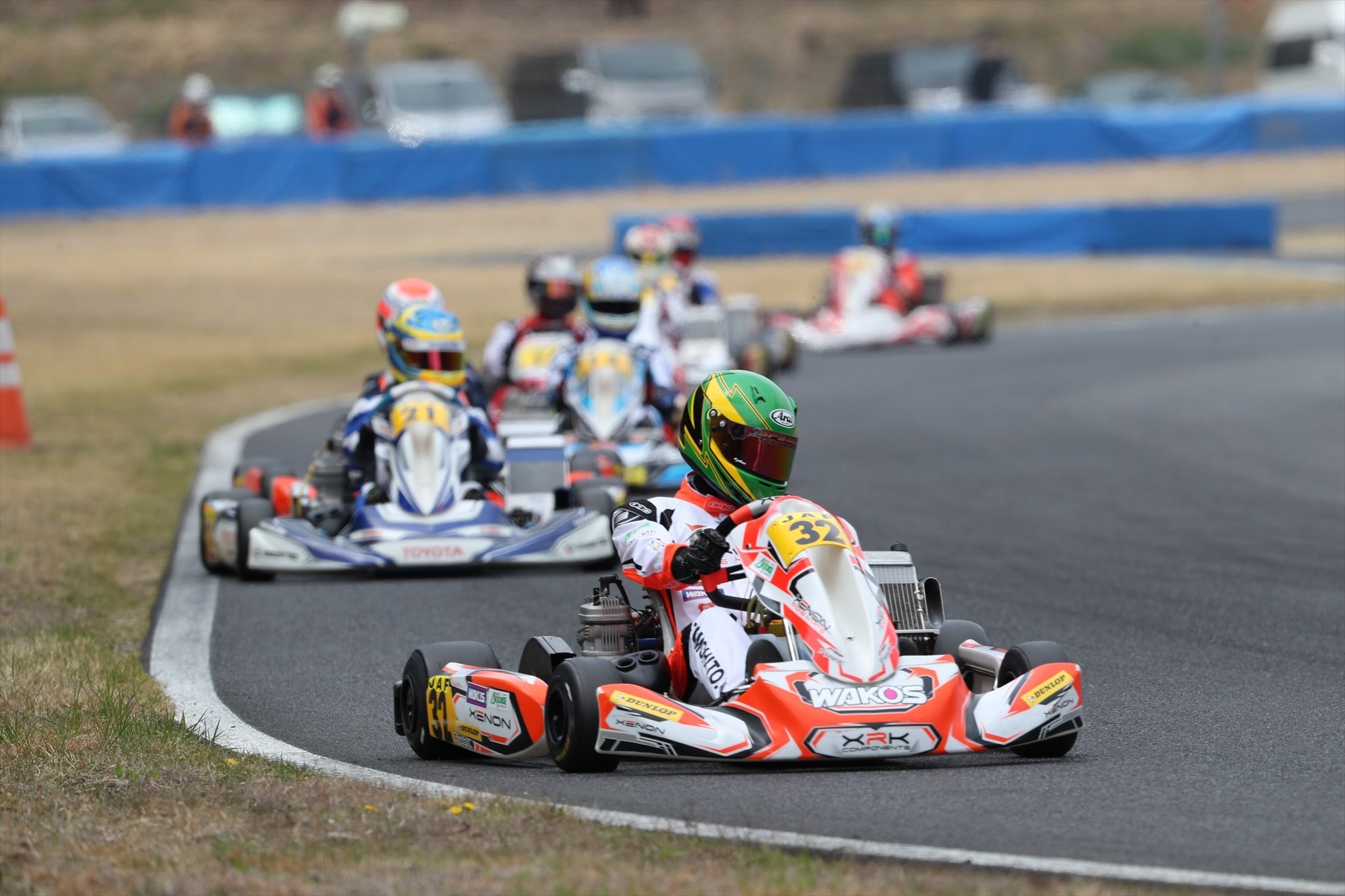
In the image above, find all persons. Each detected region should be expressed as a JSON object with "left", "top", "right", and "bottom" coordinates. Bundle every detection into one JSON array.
[
  {"left": 342, "top": 279, "right": 504, "bottom": 516},
  {"left": 857, "top": 202, "right": 923, "bottom": 318},
  {"left": 609, "top": 370, "right": 918, "bottom": 703},
  {"left": 832, "top": 17, "right": 1023, "bottom": 115},
  {"left": 165, "top": 74, "right": 217, "bottom": 140},
  {"left": 303, "top": 62, "right": 356, "bottom": 143},
  {"left": 483, "top": 214, "right": 723, "bottom": 481}
]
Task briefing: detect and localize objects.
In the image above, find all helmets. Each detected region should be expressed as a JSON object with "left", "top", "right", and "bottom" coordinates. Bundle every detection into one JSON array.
[
  {"left": 374, "top": 277, "right": 447, "bottom": 349},
  {"left": 623, "top": 223, "right": 675, "bottom": 265},
  {"left": 666, "top": 217, "right": 698, "bottom": 252},
  {"left": 579, "top": 254, "right": 641, "bottom": 334},
  {"left": 526, "top": 252, "right": 581, "bottom": 319},
  {"left": 856, "top": 209, "right": 894, "bottom": 251},
  {"left": 678, "top": 370, "right": 799, "bottom": 506},
  {"left": 387, "top": 303, "right": 468, "bottom": 388}
]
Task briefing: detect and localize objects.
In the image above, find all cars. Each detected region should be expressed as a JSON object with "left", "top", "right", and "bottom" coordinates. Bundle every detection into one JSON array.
[
  {"left": 364, "top": 55, "right": 516, "bottom": 146},
  {"left": 828, "top": 37, "right": 1060, "bottom": 113},
  {"left": 500, "top": 30, "right": 725, "bottom": 126},
  {"left": 0, "top": 92, "right": 138, "bottom": 168}
]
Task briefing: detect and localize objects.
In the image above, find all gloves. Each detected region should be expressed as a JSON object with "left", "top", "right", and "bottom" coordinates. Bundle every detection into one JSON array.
[{"left": 670, "top": 527, "right": 733, "bottom": 584}]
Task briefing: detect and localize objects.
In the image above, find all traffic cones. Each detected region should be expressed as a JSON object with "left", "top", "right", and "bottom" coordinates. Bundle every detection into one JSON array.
[{"left": 1, "top": 294, "right": 37, "bottom": 449}]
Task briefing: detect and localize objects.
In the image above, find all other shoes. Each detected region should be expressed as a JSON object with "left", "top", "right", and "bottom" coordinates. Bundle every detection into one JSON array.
[
  {"left": 745, "top": 640, "right": 784, "bottom": 683},
  {"left": 464, "top": 489, "right": 483, "bottom": 499},
  {"left": 365, "top": 486, "right": 388, "bottom": 505},
  {"left": 897, "top": 636, "right": 917, "bottom": 656}
]
